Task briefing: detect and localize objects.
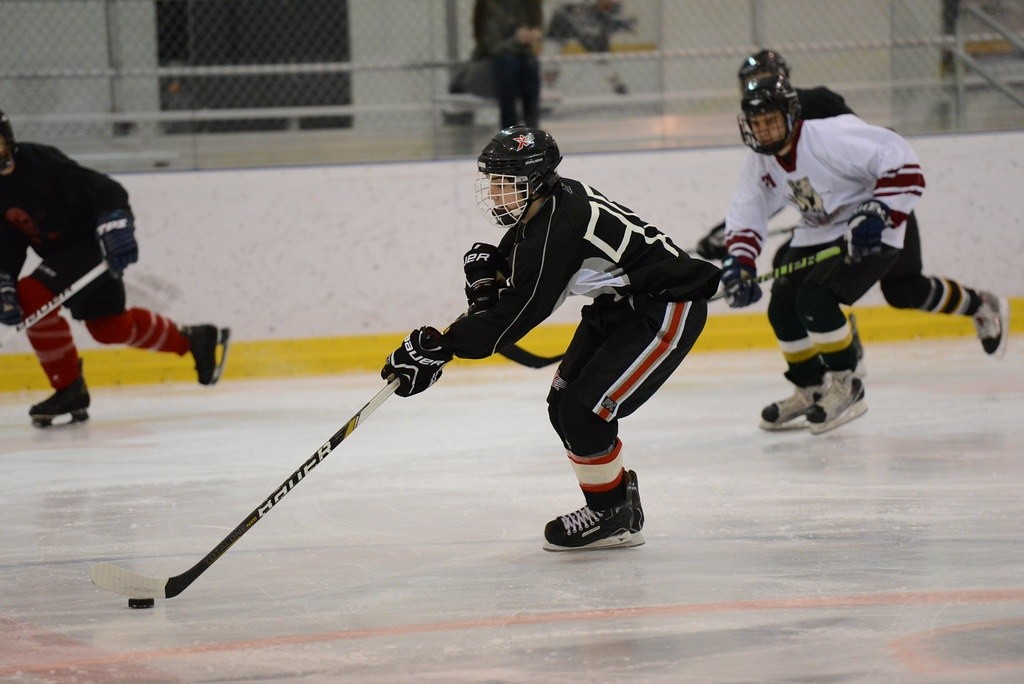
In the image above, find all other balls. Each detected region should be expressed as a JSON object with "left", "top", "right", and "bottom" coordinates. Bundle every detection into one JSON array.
[{"left": 127, "top": 597, "right": 155, "bottom": 609}]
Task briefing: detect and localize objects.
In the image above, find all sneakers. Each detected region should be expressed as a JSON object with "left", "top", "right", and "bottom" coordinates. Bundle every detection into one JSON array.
[
  {"left": 543, "top": 470, "right": 644, "bottom": 551},
  {"left": 974, "top": 291, "right": 1007, "bottom": 359},
  {"left": 759, "top": 385, "right": 812, "bottom": 430},
  {"left": 182, "top": 323, "right": 229, "bottom": 385},
  {"left": 29, "top": 378, "right": 90, "bottom": 427},
  {"left": 805, "top": 371, "right": 867, "bottom": 434}
]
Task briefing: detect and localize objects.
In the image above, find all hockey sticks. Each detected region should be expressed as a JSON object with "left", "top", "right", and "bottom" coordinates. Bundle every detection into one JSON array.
[
  {"left": 678, "top": 224, "right": 802, "bottom": 255},
  {"left": 89, "top": 374, "right": 402, "bottom": 598},
  {"left": 0, "top": 262, "right": 109, "bottom": 351},
  {"left": 498, "top": 243, "right": 848, "bottom": 370}
]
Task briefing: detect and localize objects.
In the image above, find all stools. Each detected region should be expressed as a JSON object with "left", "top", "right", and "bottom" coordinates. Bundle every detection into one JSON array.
[{"left": 435, "top": 91, "right": 564, "bottom": 156}]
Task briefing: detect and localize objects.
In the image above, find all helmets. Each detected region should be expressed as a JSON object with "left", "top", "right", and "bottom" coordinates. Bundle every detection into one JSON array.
[
  {"left": 739, "top": 49, "right": 788, "bottom": 79},
  {"left": 741, "top": 81, "right": 802, "bottom": 155},
  {"left": 0, "top": 111, "right": 15, "bottom": 171},
  {"left": 478, "top": 124, "right": 562, "bottom": 225}
]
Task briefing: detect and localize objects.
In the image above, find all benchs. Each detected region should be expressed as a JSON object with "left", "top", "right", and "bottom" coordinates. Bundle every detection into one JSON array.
[{"left": 57, "top": 142, "right": 178, "bottom": 170}]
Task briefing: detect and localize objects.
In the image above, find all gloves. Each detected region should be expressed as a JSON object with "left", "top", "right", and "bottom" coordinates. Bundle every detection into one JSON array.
[
  {"left": 462, "top": 243, "right": 509, "bottom": 299},
  {"left": 836, "top": 200, "right": 891, "bottom": 264},
  {"left": 0, "top": 272, "right": 20, "bottom": 324},
  {"left": 98, "top": 227, "right": 137, "bottom": 279},
  {"left": 722, "top": 257, "right": 762, "bottom": 308},
  {"left": 381, "top": 326, "right": 451, "bottom": 397}
]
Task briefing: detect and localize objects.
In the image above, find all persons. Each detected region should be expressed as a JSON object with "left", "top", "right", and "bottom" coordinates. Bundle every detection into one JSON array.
[
  {"left": 463, "top": 0, "right": 545, "bottom": 130},
  {"left": 381, "top": 125, "right": 723, "bottom": 551},
  {"left": 0, "top": 108, "right": 231, "bottom": 430},
  {"left": 541, "top": 1, "right": 640, "bottom": 103},
  {"left": 699, "top": 47, "right": 1008, "bottom": 434}
]
